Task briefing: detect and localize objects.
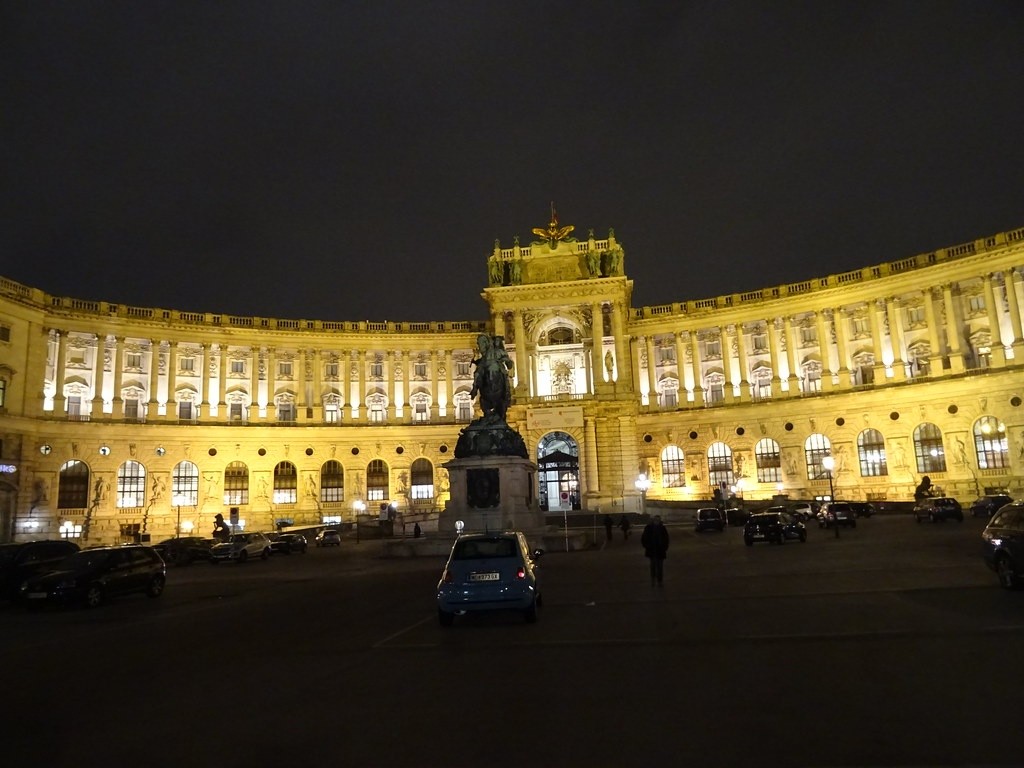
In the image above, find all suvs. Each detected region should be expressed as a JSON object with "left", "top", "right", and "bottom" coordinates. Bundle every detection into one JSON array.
[
  {"left": 208, "top": 533, "right": 271, "bottom": 565},
  {"left": 978, "top": 500, "right": 1024, "bottom": 589},
  {"left": 17, "top": 544, "right": 167, "bottom": 608},
  {"left": 914, "top": 497, "right": 961, "bottom": 523},
  {"left": 817, "top": 503, "right": 856, "bottom": 529},
  {"left": 970, "top": 495, "right": 1014, "bottom": 518},
  {"left": 0, "top": 542, "right": 81, "bottom": 607}
]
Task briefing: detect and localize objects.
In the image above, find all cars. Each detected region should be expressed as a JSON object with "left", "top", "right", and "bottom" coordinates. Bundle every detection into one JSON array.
[
  {"left": 315, "top": 530, "right": 341, "bottom": 546},
  {"left": 269, "top": 534, "right": 308, "bottom": 554},
  {"left": 693, "top": 508, "right": 725, "bottom": 531},
  {"left": 852, "top": 501, "right": 875, "bottom": 516},
  {"left": 788, "top": 501, "right": 815, "bottom": 520},
  {"left": 744, "top": 512, "right": 807, "bottom": 545},
  {"left": 435, "top": 530, "right": 543, "bottom": 625}
]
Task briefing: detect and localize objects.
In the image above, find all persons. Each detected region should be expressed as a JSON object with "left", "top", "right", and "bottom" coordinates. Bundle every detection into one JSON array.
[
  {"left": 603, "top": 514, "right": 613, "bottom": 541},
  {"left": 414, "top": 523, "right": 421, "bottom": 538},
  {"left": 641, "top": 515, "right": 669, "bottom": 585},
  {"left": 617, "top": 515, "right": 630, "bottom": 541},
  {"left": 468, "top": 334, "right": 511, "bottom": 423}
]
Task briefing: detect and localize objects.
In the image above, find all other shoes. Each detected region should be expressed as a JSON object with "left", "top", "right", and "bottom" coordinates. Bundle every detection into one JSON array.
[
  {"left": 657, "top": 580, "right": 664, "bottom": 587},
  {"left": 652, "top": 579, "right": 656, "bottom": 586}
]
